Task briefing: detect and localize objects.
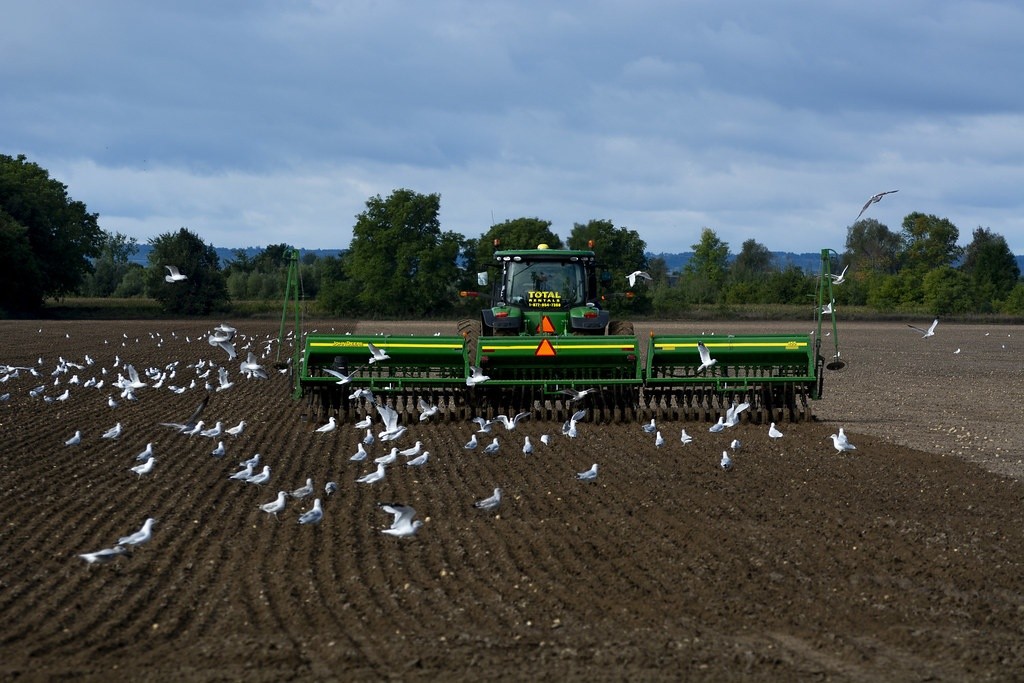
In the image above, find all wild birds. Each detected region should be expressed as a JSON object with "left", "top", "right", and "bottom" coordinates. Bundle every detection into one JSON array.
[
  {"left": 164, "top": 264, "right": 189, "bottom": 284},
  {"left": 654, "top": 431, "right": 664, "bottom": 452},
  {"left": 985, "top": 332, "right": 990, "bottom": 336},
  {"left": 626, "top": 269, "right": 654, "bottom": 288},
  {"left": 815, "top": 297, "right": 837, "bottom": 315},
  {"left": 810, "top": 330, "right": 814, "bottom": 334},
  {"left": 827, "top": 426, "right": 858, "bottom": 456},
  {"left": 1002, "top": 344, "right": 1005, "bottom": 349},
  {"left": 731, "top": 438, "right": 740, "bottom": 455},
  {"left": 953, "top": 348, "right": 961, "bottom": 354},
  {"left": 1007, "top": 334, "right": 1011, "bottom": 338},
  {"left": 680, "top": 429, "right": 694, "bottom": 448},
  {"left": 696, "top": 340, "right": 719, "bottom": 373},
  {"left": 641, "top": 418, "right": 656, "bottom": 435},
  {"left": 0, "top": 322, "right": 534, "bottom": 575},
  {"left": 708, "top": 399, "right": 755, "bottom": 433},
  {"left": 768, "top": 422, "right": 784, "bottom": 443},
  {"left": 854, "top": 189, "right": 900, "bottom": 221},
  {"left": 571, "top": 462, "right": 599, "bottom": 487},
  {"left": 813, "top": 264, "right": 849, "bottom": 285},
  {"left": 720, "top": 450, "right": 734, "bottom": 473},
  {"left": 905, "top": 315, "right": 941, "bottom": 340},
  {"left": 824, "top": 333, "right": 831, "bottom": 337},
  {"left": 539, "top": 385, "right": 597, "bottom": 447},
  {"left": 833, "top": 351, "right": 841, "bottom": 358}
]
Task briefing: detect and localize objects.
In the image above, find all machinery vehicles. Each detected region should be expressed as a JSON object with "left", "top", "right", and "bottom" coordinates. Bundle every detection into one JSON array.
[{"left": 272, "top": 234, "right": 847, "bottom": 425}]
